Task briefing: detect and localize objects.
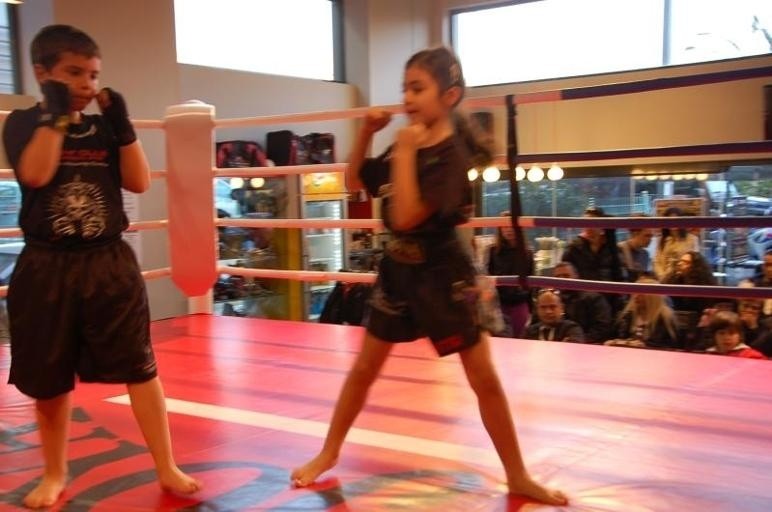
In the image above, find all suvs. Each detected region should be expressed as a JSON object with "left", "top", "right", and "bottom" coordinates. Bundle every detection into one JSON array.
[{"left": 672, "top": 177, "right": 771, "bottom": 221}]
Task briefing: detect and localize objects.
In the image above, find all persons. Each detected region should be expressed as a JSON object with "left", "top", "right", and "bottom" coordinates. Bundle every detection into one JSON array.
[
  {"left": 289, "top": 47, "right": 568, "bottom": 508},
  {"left": 3, "top": 23, "right": 201, "bottom": 510},
  {"left": 322, "top": 207, "right": 771, "bottom": 358}
]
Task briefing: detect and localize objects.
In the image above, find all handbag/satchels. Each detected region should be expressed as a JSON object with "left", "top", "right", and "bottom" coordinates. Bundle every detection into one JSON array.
[
  {"left": 217, "top": 141, "right": 269, "bottom": 167},
  {"left": 268, "top": 131, "right": 334, "bottom": 166}
]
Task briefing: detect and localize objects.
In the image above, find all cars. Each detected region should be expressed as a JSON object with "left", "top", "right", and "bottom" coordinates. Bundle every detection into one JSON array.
[
  {"left": 214, "top": 177, "right": 245, "bottom": 220},
  {"left": 0, "top": 180, "right": 28, "bottom": 316}
]
